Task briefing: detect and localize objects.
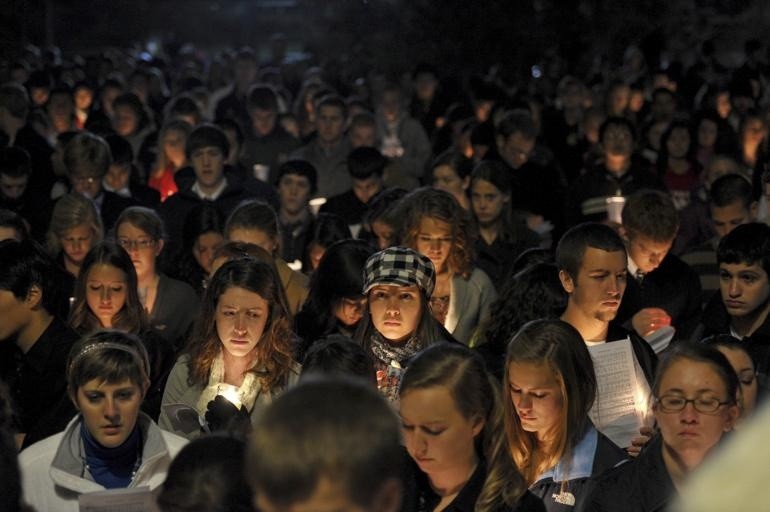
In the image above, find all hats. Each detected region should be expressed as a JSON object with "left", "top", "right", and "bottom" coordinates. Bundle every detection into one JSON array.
[{"left": 362, "top": 246, "right": 435, "bottom": 300}]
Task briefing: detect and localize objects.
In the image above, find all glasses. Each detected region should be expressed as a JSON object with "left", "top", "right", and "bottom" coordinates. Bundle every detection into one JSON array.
[{"left": 655, "top": 395, "right": 731, "bottom": 413}]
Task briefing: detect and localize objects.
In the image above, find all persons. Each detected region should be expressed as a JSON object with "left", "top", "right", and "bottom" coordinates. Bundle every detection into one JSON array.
[{"left": 1, "top": 17, "right": 769, "bottom": 512}]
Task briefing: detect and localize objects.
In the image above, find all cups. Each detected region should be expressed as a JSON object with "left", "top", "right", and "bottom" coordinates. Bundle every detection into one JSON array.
[{"left": 216, "top": 384, "right": 244, "bottom": 410}]
